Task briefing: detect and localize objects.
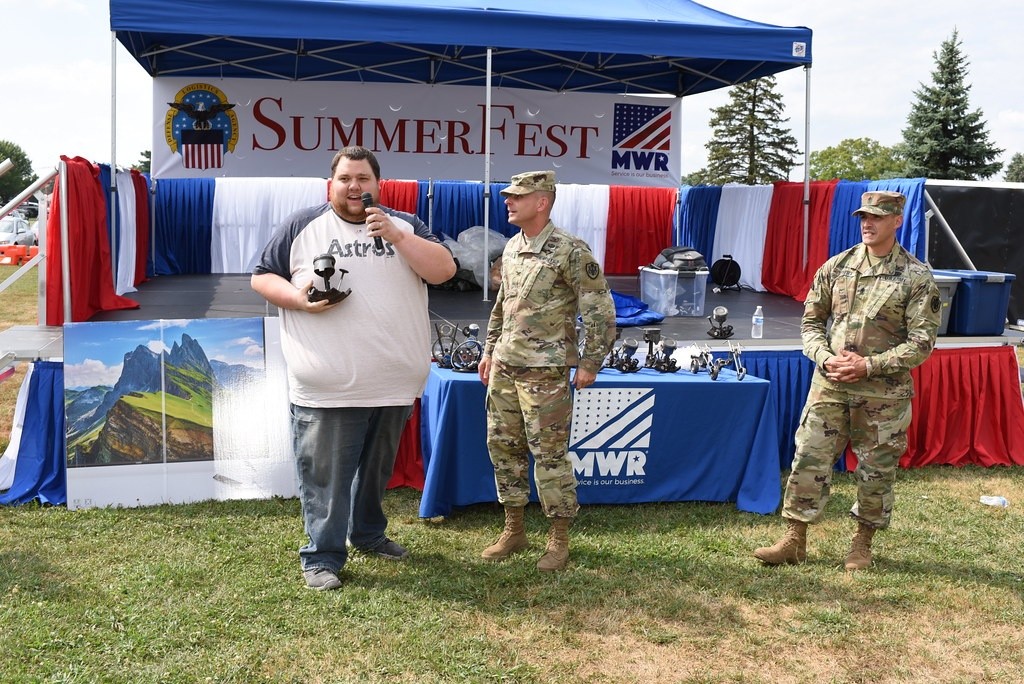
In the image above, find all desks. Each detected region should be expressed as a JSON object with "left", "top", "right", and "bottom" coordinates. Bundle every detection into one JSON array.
[
  {"left": 710, "top": 342, "right": 1017, "bottom": 460},
  {"left": 425, "top": 362, "right": 771, "bottom": 500}
]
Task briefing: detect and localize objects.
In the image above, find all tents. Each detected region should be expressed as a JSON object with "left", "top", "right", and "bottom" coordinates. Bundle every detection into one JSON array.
[{"left": 109, "top": 0, "right": 813, "bottom": 301}]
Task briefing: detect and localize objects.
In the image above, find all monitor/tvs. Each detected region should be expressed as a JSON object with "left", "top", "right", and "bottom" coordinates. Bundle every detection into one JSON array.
[{"left": 63, "top": 316, "right": 268, "bottom": 472}]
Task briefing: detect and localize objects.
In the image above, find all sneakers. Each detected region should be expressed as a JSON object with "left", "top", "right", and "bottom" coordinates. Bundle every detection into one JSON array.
[
  {"left": 303, "top": 569, "right": 342, "bottom": 591},
  {"left": 373, "top": 538, "right": 410, "bottom": 559}
]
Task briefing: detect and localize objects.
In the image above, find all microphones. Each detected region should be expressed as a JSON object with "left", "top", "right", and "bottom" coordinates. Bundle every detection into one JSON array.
[{"left": 362, "top": 192, "right": 384, "bottom": 250}]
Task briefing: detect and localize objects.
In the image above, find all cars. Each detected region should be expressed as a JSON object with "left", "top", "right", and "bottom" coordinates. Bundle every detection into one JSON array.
[
  {"left": 32, "top": 220, "right": 39, "bottom": 235},
  {"left": 0, "top": 200, "right": 38, "bottom": 219},
  {"left": 0, "top": 216, "right": 38, "bottom": 246}
]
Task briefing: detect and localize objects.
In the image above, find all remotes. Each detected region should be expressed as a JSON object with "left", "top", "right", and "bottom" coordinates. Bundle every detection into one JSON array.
[{"left": 214, "top": 474, "right": 243, "bottom": 488}]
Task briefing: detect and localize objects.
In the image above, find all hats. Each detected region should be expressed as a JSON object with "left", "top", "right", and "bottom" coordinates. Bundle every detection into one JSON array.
[
  {"left": 852, "top": 190, "right": 905, "bottom": 216},
  {"left": 499, "top": 170, "right": 556, "bottom": 196}
]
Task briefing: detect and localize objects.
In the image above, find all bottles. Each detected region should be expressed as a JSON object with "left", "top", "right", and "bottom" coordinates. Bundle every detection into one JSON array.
[
  {"left": 979, "top": 495, "right": 1010, "bottom": 509},
  {"left": 751, "top": 306, "right": 763, "bottom": 339}
]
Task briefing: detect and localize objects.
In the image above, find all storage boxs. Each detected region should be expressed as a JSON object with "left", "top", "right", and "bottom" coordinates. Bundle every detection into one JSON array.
[
  {"left": 930, "top": 269, "right": 1016, "bottom": 336},
  {"left": 638, "top": 266, "right": 710, "bottom": 316}
]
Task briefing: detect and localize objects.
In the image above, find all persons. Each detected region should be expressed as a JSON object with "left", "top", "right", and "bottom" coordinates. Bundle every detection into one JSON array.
[
  {"left": 250, "top": 146, "right": 457, "bottom": 591},
  {"left": 31, "top": 208, "right": 50, "bottom": 246},
  {"left": 478, "top": 171, "right": 616, "bottom": 571},
  {"left": 754, "top": 191, "right": 942, "bottom": 571}
]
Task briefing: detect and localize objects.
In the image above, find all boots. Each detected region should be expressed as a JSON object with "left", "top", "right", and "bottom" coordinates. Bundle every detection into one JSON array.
[
  {"left": 845, "top": 522, "right": 876, "bottom": 569},
  {"left": 537, "top": 518, "right": 570, "bottom": 570},
  {"left": 754, "top": 520, "right": 807, "bottom": 562},
  {"left": 481, "top": 506, "right": 529, "bottom": 559}
]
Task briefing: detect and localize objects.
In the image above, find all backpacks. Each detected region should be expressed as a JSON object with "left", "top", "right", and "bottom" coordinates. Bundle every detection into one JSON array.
[{"left": 654, "top": 247, "right": 708, "bottom": 270}]
